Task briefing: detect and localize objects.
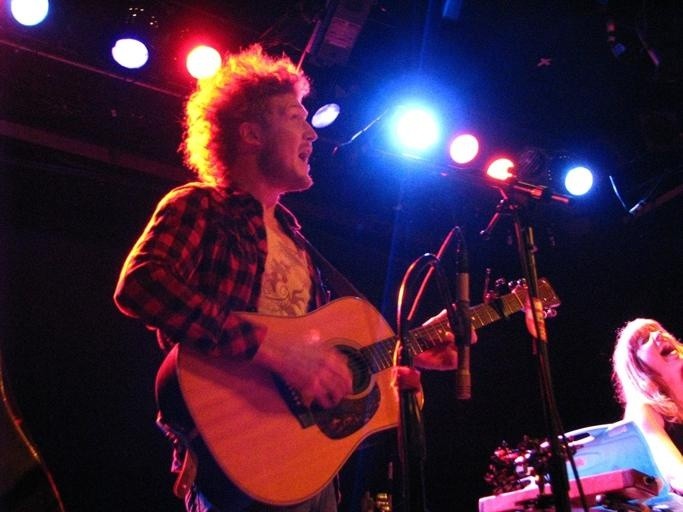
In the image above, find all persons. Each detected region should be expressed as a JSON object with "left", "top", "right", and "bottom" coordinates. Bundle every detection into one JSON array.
[
  {"left": 111, "top": 40, "right": 481, "bottom": 509},
  {"left": 610, "top": 309, "right": 682, "bottom": 492}
]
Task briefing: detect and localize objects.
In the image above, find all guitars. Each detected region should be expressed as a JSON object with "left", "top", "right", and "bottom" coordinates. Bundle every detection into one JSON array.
[{"left": 175, "top": 278, "right": 561, "bottom": 504}]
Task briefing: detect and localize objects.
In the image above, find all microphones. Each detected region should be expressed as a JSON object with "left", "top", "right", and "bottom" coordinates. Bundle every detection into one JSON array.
[
  {"left": 333, "top": 110, "right": 388, "bottom": 162},
  {"left": 455, "top": 245, "right": 472, "bottom": 399}
]
